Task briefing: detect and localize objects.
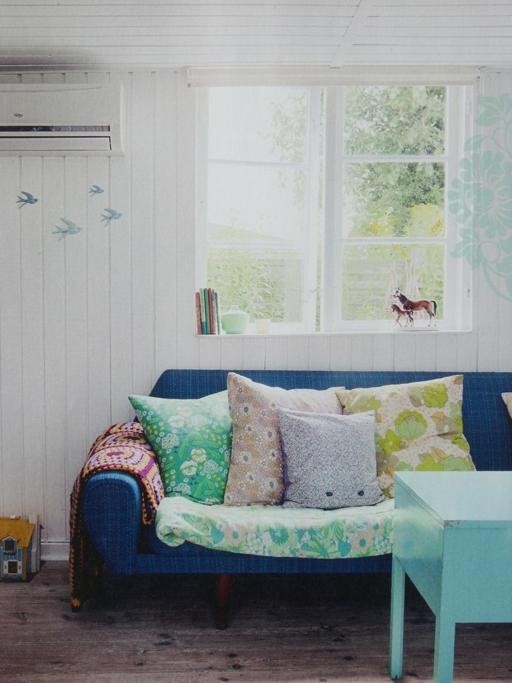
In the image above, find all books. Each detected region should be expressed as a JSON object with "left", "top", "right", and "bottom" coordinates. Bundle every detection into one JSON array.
[{"left": 195, "top": 287, "right": 220, "bottom": 333}]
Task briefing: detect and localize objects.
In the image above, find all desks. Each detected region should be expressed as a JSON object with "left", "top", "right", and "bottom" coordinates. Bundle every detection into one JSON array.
[{"left": 388, "top": 470, "right": 512, "bottom": 682}]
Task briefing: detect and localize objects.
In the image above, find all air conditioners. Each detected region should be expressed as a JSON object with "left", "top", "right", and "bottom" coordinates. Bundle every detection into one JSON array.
[{"left": 0, "top": 64, "right": 120, "bottom": 156}]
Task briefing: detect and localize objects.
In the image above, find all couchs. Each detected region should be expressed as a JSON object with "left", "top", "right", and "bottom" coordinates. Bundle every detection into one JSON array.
[{"left": 69, "top": 368, "right": 511, "bottom": 630}]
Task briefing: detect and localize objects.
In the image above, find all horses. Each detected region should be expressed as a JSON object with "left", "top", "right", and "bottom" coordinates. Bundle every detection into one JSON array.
[
  {"left": 391, "top": 303, "right": 413, "bottom": 326},
  {"left": 393, "top": 287, "right": 437, "bottom": 327}
]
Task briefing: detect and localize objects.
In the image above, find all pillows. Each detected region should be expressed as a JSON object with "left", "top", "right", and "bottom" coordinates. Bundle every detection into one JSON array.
[
  {"left": 277, "top": 405, "right": 385, "bottom": 508},
  {"left": 334, "top": 374, "right": 474, "bottom": 495},
  {"left": 223, "top": 371, "right": 343, "bottom": 505},
  {"left": 128, "top": 391, "right": 233, "bottom": 504}
]
